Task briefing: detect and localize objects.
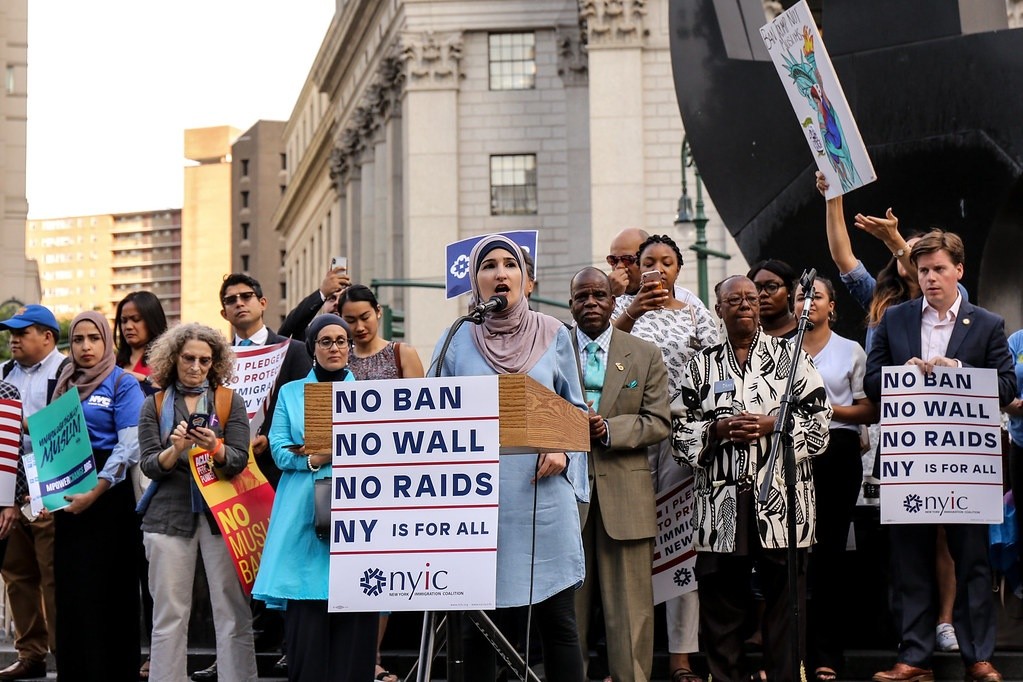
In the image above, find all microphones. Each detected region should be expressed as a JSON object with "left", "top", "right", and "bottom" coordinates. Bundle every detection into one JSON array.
[{"left": 474, "top": 294, "right": 508, "bottom": 315}]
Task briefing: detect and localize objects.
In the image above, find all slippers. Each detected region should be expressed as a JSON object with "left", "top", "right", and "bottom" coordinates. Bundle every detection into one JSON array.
[{"left": 673, "top": 668, "right": 703, "bottom": 682}]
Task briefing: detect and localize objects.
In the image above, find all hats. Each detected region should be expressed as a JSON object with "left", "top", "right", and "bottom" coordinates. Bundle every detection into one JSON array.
[
  {"left": 0, "top": 305, "right": 59, "bottom": 332},
  {"left": 305, "top": 314, "right": 351, "bottom": 359}
]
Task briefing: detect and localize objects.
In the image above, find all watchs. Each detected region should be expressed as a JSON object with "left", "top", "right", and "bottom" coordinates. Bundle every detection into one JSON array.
[{"left": 893, "top": 243, "right": 912, "bottom": 258}]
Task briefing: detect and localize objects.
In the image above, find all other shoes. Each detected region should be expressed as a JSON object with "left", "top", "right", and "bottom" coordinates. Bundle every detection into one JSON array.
[{"left": 139, "top": 658, "right": 150, "bottom": 677}]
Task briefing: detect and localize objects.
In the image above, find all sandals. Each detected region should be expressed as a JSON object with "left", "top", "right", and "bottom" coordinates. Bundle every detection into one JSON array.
[
  {"left": 816, "top": 666, "right": 836, "bottom": 682},
  {"left": 374, "top": 664, "right": 401, "bottom": 682}
]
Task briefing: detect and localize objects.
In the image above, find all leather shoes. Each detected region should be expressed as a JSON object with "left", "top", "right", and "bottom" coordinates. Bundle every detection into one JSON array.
[
  {"left": 192, "top": 659, "right": 219, "bottom": 682},
  {"left": 965, "top": 660, "right": 1002, "bottom": 682},
  {"left": 274, "top": 654, "right": 287, "bottom": 667},
  {"left": 0, "top": 658, "right": 47, "bottom": 678},
  {"left": 872, "top": 662, "right": 935, "bottom": 682}
]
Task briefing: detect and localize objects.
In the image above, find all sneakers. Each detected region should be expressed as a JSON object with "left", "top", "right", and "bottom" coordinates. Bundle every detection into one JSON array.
[{"left": 935, "top": 623, "right": 960, "bottom": 650}]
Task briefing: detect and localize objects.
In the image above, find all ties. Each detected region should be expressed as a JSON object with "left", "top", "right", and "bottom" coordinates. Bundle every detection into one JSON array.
[{"left": 586, "top": 343, "right": 604, "bottom": 413}]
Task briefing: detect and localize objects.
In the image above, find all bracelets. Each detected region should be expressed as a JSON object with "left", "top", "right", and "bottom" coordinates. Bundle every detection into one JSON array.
[
  {"left": 144, "top": 377, "right": 154, "bottom": 384},
  {"left": 308, "top": 454, "right": 321, "bottom": 471},
  {"left": 207, "top": 439, "right": 222, "bottom": 455},
  {"left": 625, "top": 309, "right": 637, "bottom": 321}
]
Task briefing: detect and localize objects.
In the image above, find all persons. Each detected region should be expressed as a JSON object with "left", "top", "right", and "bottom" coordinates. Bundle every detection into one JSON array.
[
  {"left": 744, "top": 171, "right": 1023, "bottom": 682},
  {"left": 0, "top": 304, "right": 71, "bottom": 682},
  {"left": 607, "top": 227, "right": 706, "bottom": 308},
  {"left": 277, "top": 265, "right": 351, "bottom": 342},
  {"left": 427, "top": 235, "right": 589, "bottom": 682},
  {"left": 670, "top": 275, "right": 833, "bottom": 682},
  {"left": 613, "top": 234, "right": 719, "bottom": 682},
  {"left": 337, "top": 285, "right": 425, "bottom": 682},
  {"left": 251, "top": 314, "right": 381, "bottom": 682},
  {"left": 568, "top": 267, "right": 671, "bottom": 682},
  {"left": 26, "top": 311, "right": 144, "bottom": 682},
  {"left": 112, "top": 291, "right": 167, "bottom": 676},
  {"left": 194, "top": 274, "right": 310, "bottom": 682},
  {"left": 137, "top": 325, "right": 258, "bottom": 682}
]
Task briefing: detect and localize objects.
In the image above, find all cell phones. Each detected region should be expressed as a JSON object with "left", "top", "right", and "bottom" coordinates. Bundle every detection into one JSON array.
[
  {"left": 331, "top": 256, "right": 347, "bottom": 289},
  {"left": 641, "top": 271, "right": 663, "bottom": 307},
  {"left": 281, "top": 444, "right": 303, "bottom": 450},
  {"left": 184, "top": 413, "right": 207, "bottom": 439},
  {"left": 20, "top": 502, "right": 37, "bottom": 523}
]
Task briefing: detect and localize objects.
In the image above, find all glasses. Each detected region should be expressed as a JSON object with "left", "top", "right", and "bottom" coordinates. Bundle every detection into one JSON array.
[
  {"left": 222, "top": 291, "right": 259, "bottom": 305},
  {"left": 719, "top": 294, "right": 760, "bottom": 306},
  {"left": 179, "top": 353, "right": 214, "bottom": 366},
  {"left": 316, "top": 336, "right": 352, "bottom": 349},
  {"left": 606, "top": 254, "right": 639, "bottom": 267},
  {"left": 756, "top": 282, "right": 786, "bottom": 295}
]
